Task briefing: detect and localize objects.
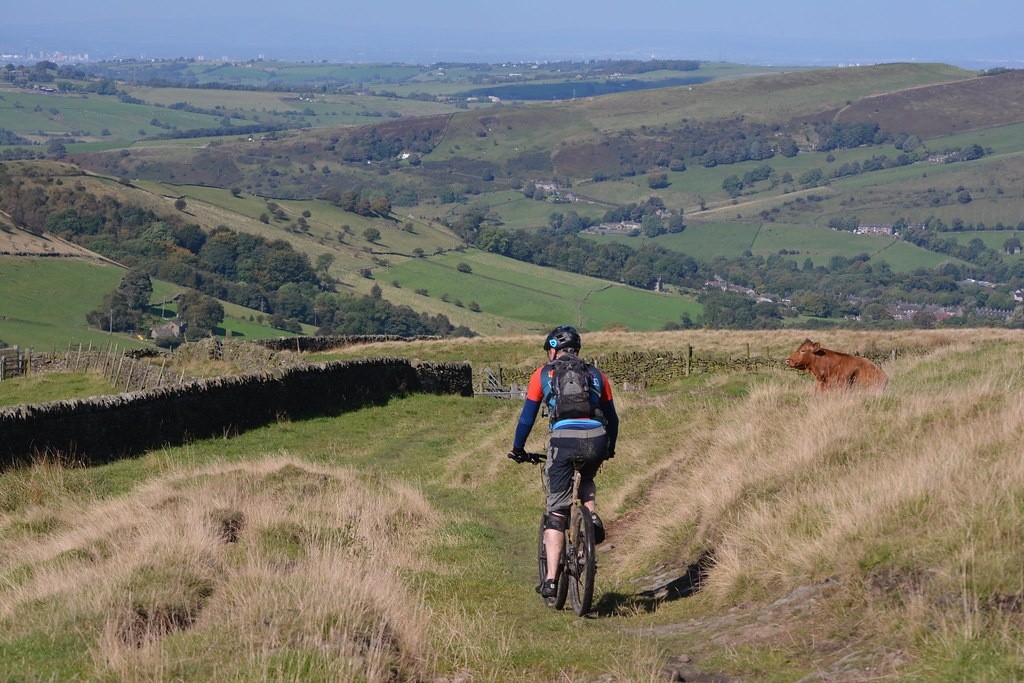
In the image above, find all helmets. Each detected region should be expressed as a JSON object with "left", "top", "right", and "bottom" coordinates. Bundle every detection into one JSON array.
[{"left": 544, "top": 325, "right": 581, "bottom": 351}]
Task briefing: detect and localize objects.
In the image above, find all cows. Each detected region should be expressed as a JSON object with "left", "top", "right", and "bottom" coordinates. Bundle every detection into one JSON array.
[{"left": 785, "top": 338, "right": 889, "bottom": 398}]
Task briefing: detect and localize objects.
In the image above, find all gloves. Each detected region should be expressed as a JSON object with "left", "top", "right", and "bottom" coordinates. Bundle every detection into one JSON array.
[{"left": 512, "top": 446, "right": 528, "bottom": 463}]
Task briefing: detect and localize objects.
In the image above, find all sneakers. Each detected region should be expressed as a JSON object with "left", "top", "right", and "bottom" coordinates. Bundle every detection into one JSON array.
[
  {"left": 534, "top": 578, "right": 557, "bottom": 597},
  {"left": 591, "top": 511, "right": 605, "bottom": 544}
]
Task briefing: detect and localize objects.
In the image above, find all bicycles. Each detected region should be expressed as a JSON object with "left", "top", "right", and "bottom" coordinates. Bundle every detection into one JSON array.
[{"left": 507, "top": 451, "right": 604, "bottom": 617}]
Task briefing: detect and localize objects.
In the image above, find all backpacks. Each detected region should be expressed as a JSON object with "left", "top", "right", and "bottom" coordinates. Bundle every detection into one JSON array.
[{"left": 547, "top": 354, "right": 602, "bottom": 421}]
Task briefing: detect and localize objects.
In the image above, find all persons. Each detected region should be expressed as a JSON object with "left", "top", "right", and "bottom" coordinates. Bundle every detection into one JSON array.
[{"left": 512, "top": 324, "right": 620, "bottom": 598}]
